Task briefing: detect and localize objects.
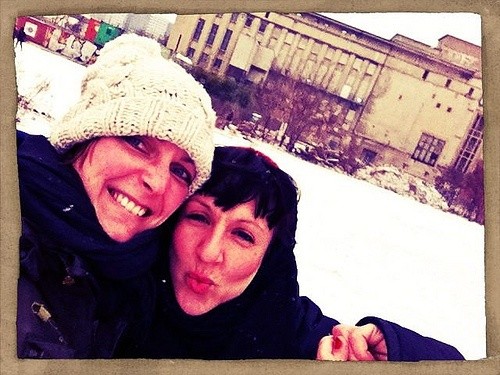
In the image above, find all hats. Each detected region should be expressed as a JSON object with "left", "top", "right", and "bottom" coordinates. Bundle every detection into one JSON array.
[{"left": 48, "top": 32, "right": 216, "bottom": 201}]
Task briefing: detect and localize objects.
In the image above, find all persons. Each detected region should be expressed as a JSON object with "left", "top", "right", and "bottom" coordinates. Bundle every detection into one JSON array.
[
  {"left": 116, "top": 146, "right": 466, "bottom": 361},
  {"left": 16, "top": 33, "right": 216, "bottom": 360},
  {"left": 222, "top": 110, "right": 234, "bottom": 129},
  {"left": 14, "top": 27, "right": 27, "bottom": 52}
]
